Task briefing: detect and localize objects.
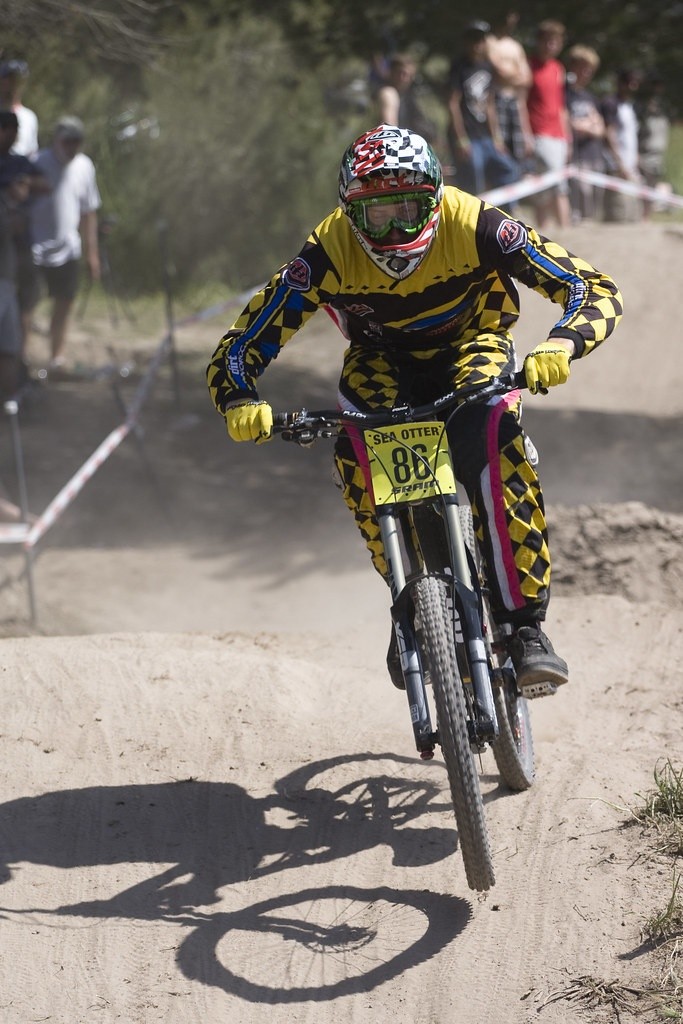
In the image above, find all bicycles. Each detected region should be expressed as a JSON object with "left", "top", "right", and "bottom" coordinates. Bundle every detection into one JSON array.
[{"left": 277, "top": 369, "right": 549, "bottom": 893}]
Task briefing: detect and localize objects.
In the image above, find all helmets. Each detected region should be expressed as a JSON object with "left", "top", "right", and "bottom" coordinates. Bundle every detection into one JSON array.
[{"left": 336, "top": 122, "right": 444, "bottom": 281}]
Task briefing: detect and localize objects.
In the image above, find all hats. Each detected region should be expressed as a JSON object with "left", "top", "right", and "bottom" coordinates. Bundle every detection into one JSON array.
[
  {"left": 459, "top": 18, "right": 492, "bottom": 36},
  {"left": 45, "top": 115, "right": 85, "bottom": 140}
]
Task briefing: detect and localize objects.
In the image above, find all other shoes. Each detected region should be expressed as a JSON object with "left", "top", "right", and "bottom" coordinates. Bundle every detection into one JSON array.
[
  {"left": 535, "top": 223, "right": 585, "bottom": 240},
  {"left": 18, "top": 361, "right": 29, "bottom": 385},
  {"left": 48, "top": 355, "right": 64, "bottom": 369}
]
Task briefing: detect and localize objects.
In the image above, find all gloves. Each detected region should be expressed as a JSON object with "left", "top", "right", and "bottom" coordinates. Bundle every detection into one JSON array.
[
  {"left": 521, "top": 342, "right": 573, "bottom": 395},
  {"left": 225, "top": 396, "right": 274, "bottom": 445}
]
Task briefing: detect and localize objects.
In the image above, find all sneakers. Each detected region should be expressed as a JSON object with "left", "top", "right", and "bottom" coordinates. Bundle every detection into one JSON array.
[
  {"left": 386, "top": 605, "right": 432, "bottom": 690},
  {"left": 509, "top": 618, "right": 568, "bottom": 692}
]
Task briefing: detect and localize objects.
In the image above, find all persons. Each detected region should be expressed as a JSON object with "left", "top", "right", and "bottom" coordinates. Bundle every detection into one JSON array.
[
  {"left": 3, "top": 113, "right": 56, "bottom": 406},
  {"left": 523, "top": 21, "right": 574, "bottom": 227},
  {"left": 445, "top": 24, "right": 515, "bottom": 215},
  {"left": 596, "top": 61, "right": 641, "bottom": 222},
  {"left": 203, "top": 123, "right": 624, "bottom": 686},
  {"left": 1, "top": 59, "right": 47, "bottom": 367},
  {"left": 487, "top": 5, "right": 541, "bottom": 231},
  {"left": 632, "top": 77, "right": 676, "bottom": 212},
  {"left": 554, "top": 42, "right": 604, "bottom": 222},
  {"left": 358, "top": 23, "right": 424, "bottom": 131},
  {"left": 25, "top": 117, "right": 100, "bottom": 382}
]
